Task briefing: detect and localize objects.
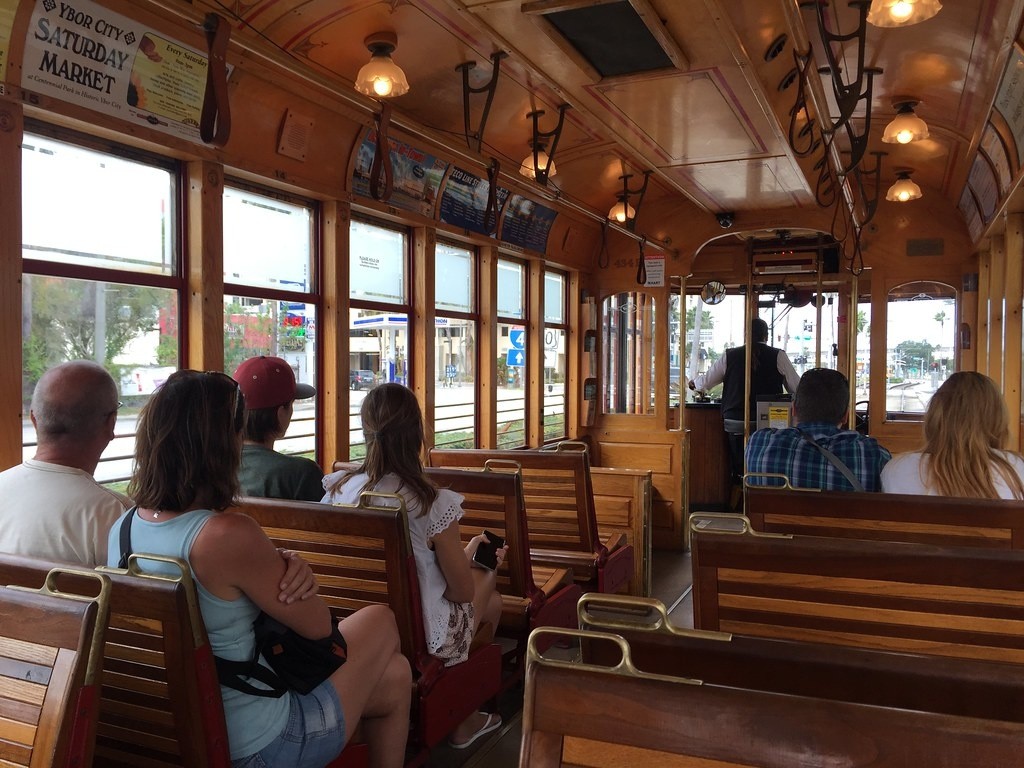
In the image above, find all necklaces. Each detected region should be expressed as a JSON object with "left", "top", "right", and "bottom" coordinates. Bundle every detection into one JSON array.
[{"left": 153, "top": 510, "right": 162, "bottom": 518}]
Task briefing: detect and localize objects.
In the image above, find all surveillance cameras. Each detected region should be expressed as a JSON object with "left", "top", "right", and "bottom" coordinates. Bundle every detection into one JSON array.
[{"left": 715, "top": 211, "right": 734, "bottom": 228}]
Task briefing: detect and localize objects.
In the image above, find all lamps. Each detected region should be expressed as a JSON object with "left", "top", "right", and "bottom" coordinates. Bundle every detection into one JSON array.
[
  {"left": 866, "top": 0, "right": 943, "bottom": 28},
  {"left": 519, "top": 137, "right": 557, "bottom": 179},
  {"left": 354, "top": 31, "right": 409, "bottom": 97},
  {"left": 881, "top": 100, "right": 931, "bottom": 144},
  {"left": 607, "top": 196, "right": 636, "bottom": 222},
  {"left": 886, "top": 167, "right": 924, "bottom": 202}
]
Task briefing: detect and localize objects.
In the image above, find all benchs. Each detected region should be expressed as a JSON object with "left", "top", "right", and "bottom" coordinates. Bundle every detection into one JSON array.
[
  {"left": 0, "top": 440, "right": 632, "bottom": 768},
  {"left": 518, "top": 471, "right": 1024, "bottom": 768}
]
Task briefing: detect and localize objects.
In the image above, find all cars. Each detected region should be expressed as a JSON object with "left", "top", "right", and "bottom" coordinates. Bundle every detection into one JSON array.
[{"left": 669, "top": 367, "right": 681, "bottom": 390}]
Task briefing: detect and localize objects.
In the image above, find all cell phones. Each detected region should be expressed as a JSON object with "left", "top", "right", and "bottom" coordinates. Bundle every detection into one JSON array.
[{"left": 473, "top": 529, "right": 506, "bottom": 571}]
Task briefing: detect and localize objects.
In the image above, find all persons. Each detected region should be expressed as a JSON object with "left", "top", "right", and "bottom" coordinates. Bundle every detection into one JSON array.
[
  {"left": 233, "top": 355, "right": 327, "bottom": 501},
  {"left": 745, "top": 367, "right": 893, "bottom": 493},
  {"left": 880, "top": 371, "right": 1024, "bottom": 501},
  {"left": 702, "top": 286, "right": 722, "bottom": 303},
  {"left": 689, "top": 319, "right": 800, "bottom": 482},
  {"left": 108, "top": 371, "right": 411, "bottom": 768},
  {"left": 323, "top": 384, "right": 508, "bottom": 749},
  {"left": 0, "top": 359, "right": 133, "bottom": 570}
]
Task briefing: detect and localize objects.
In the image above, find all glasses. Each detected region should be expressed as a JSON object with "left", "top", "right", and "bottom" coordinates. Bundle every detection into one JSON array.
[
  {"left": 107, "top": 401, "right": 124, "bottom": 425},
  {"left": 204, "top": 370, "right": 243, "bottom": 420}
]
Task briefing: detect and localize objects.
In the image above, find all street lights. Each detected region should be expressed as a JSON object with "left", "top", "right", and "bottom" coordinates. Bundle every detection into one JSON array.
[{"left": 894, "top": 347, "right": 921, "bottom": 380}]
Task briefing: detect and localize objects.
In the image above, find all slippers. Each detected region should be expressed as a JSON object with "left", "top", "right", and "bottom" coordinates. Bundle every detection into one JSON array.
[{"left": 449, "top": 712, "right": 502, "bottom": 748}]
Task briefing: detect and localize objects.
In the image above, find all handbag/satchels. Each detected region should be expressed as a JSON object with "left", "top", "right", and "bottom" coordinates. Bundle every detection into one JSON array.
[{"left": 252, "top": 606, "right": 348, "bottom": 697}]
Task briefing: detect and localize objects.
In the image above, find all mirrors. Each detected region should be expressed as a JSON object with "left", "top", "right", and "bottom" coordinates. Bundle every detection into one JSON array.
[{"left": 701, "top": 281, "right": 727, "bottom": 305}]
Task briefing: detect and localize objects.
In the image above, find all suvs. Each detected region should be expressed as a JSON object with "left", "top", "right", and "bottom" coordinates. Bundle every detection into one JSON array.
[{"left": 350, "top": 370, "right": 374, "bottom": 391}]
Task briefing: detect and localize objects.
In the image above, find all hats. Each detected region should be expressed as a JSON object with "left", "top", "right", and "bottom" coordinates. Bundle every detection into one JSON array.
[{"left": 233, "top": 356, "right": 316, "bottom": 409}]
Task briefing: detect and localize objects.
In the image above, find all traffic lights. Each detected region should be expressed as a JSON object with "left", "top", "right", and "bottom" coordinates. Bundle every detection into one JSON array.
[
  {"left": 803, "top": 324, "right": 812, "bottom": 332},
  {"left": 931, "top": 363, "right": 938, "bottom": 369},
  {"left": 795, "top": 355, "right": 808, "bottom": 365}
]
